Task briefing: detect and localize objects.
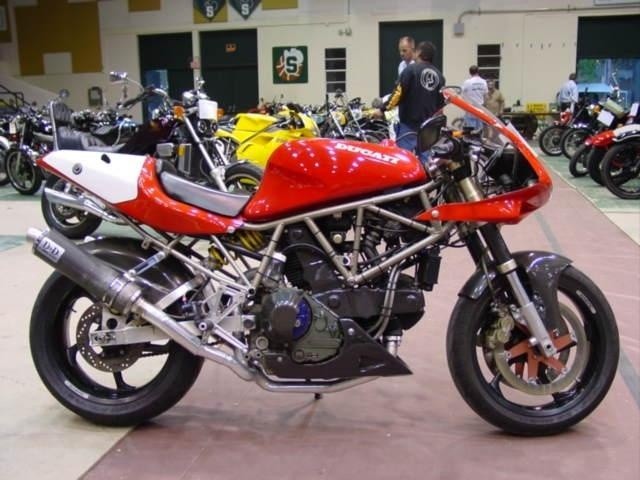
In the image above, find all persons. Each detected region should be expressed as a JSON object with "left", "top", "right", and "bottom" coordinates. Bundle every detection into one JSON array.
[
  {"left": 397, "top": 36, "right": 417, "bottom": 83},
  {"left": 555, "top": 73, "right": 585, "bottom": 123},
  {"left": 381, "top": 39, "right": 447, "bottom": 165},
  {"left": 480, "top": 74, "right": 506, "bottom": 122},
  {"left": 457, "top": 65, "right": 491, "bottom": 137}
]
